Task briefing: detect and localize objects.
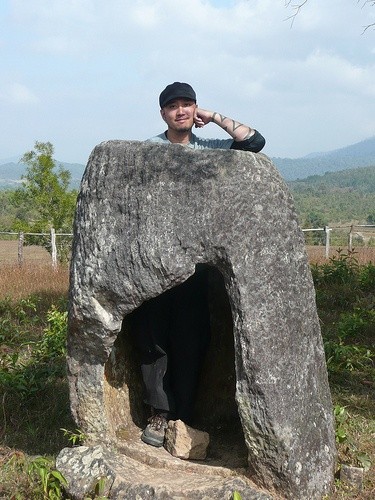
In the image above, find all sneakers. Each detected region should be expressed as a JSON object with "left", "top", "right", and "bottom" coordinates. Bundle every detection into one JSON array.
[{"left": 141, "top": 416, "right": 167, "bottom": 447}]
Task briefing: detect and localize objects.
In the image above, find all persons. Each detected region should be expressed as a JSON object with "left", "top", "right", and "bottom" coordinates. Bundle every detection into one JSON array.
[{"left": 140, "top": 82, "right": 267, "bottom": 447}]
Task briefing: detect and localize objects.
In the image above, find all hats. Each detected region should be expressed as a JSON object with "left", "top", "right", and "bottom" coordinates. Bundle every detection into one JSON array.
[{"left": 159, "top": 81, "right": 196, "bottom": 109}]
